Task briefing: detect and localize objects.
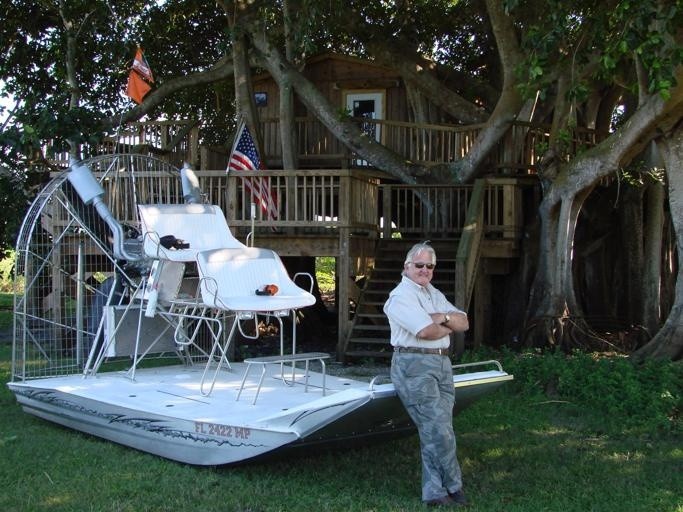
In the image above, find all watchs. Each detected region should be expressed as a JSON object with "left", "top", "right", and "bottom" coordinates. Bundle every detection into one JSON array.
[{"left": 443, "top": 313, "right": 450, "bottom": 323}]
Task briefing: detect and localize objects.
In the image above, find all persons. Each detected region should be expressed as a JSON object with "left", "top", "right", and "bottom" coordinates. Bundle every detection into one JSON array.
[{"left": 382, "top": 240, "right": 472, "bottom": 508}]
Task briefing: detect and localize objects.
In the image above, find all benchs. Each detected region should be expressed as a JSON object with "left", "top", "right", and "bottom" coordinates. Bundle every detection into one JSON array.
[
  {"left": 236, "top": 351, "right": 331, "bottom": 406},
  {"left": 130, "top": 203, "right": 316, "bottom": 321}
]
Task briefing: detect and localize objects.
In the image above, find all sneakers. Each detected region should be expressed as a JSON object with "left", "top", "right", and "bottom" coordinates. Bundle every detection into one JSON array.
[{"left": 428, "top": 491, "right": 467, "bottom": 506}]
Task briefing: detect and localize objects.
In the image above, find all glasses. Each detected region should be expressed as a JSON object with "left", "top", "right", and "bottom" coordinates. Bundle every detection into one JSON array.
[{"left": 409, "top": 262, "right": 434, "bottom": 270}]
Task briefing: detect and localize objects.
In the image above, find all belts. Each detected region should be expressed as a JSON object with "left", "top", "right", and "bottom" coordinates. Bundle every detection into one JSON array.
[{"left": 400, "top": 347, "right": 448, "bottom": 356}]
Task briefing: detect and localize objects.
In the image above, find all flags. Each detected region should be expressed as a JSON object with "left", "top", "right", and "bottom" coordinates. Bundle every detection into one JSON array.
[
  {"left": 124, "top": 48, "right": 155, "bottom": 105},
  {"left": 224, "top": 121, "right": 281, "bottom": 233}
]
